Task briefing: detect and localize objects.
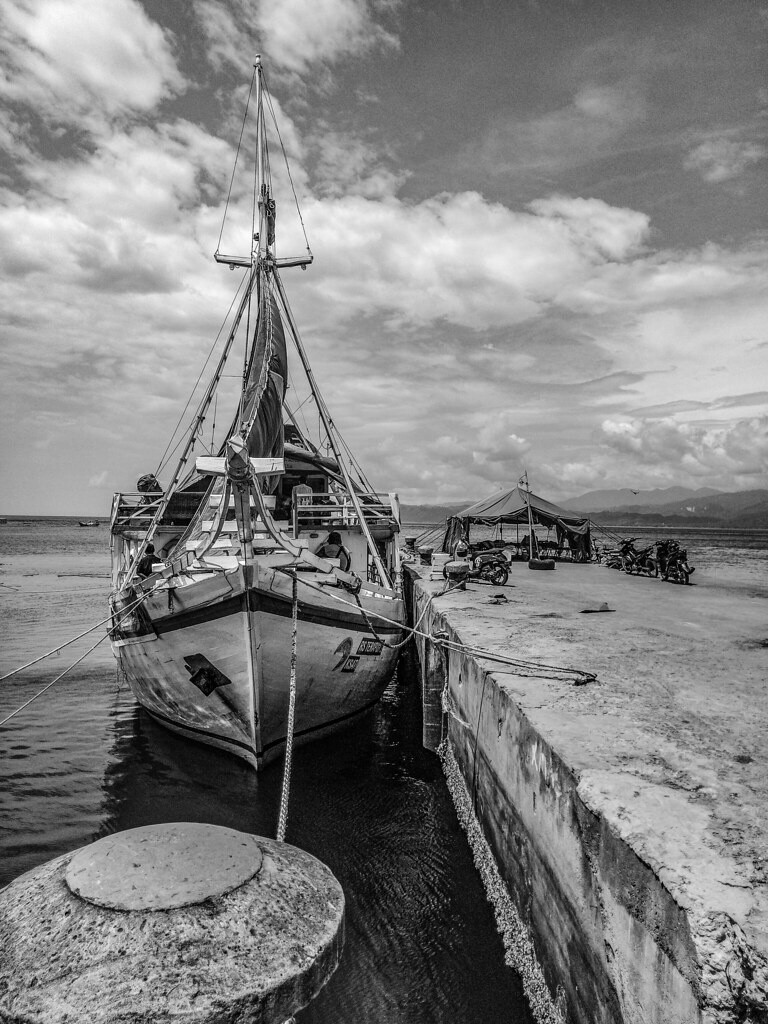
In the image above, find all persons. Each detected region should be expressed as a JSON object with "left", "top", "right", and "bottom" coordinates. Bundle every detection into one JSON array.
[
  {"left": 291, "top": 476, "right": 314, "bottom": 525},
  {"left": 314, "top": 532, "right": 351, "bottom": 573},
  {"left": 527, "top": 530, "right": 539, "bottom": 561},
  {"left": 137, "top": 543, "right": 163, "bottom": 580}
]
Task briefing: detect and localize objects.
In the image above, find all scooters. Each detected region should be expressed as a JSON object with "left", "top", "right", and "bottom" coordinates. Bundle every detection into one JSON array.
[{"left": 442, "top": 523, "right": 513, "bottom": 586}]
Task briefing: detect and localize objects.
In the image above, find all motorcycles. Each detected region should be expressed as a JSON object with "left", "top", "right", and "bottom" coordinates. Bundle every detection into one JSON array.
[
  {"left": 652, "top": 538, "right": 695, "bottom": 585},
  {"left": 617, "top": 537, "right": 658, "bottom": 578}
]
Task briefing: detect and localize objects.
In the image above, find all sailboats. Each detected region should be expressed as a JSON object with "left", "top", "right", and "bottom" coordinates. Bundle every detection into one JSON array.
[{"left": 101, "top": 52, "right": 411, "bottom": 778}]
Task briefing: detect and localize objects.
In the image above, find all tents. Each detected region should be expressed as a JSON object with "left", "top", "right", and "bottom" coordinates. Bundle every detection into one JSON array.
[{"left": 442, "top": 484, "right": 592, "bottom": 563}]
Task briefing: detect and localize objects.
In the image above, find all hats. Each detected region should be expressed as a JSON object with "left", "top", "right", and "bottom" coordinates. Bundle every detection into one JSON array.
[{"left": 326, "top": 532, "right": 343, "bottom": 544}]
